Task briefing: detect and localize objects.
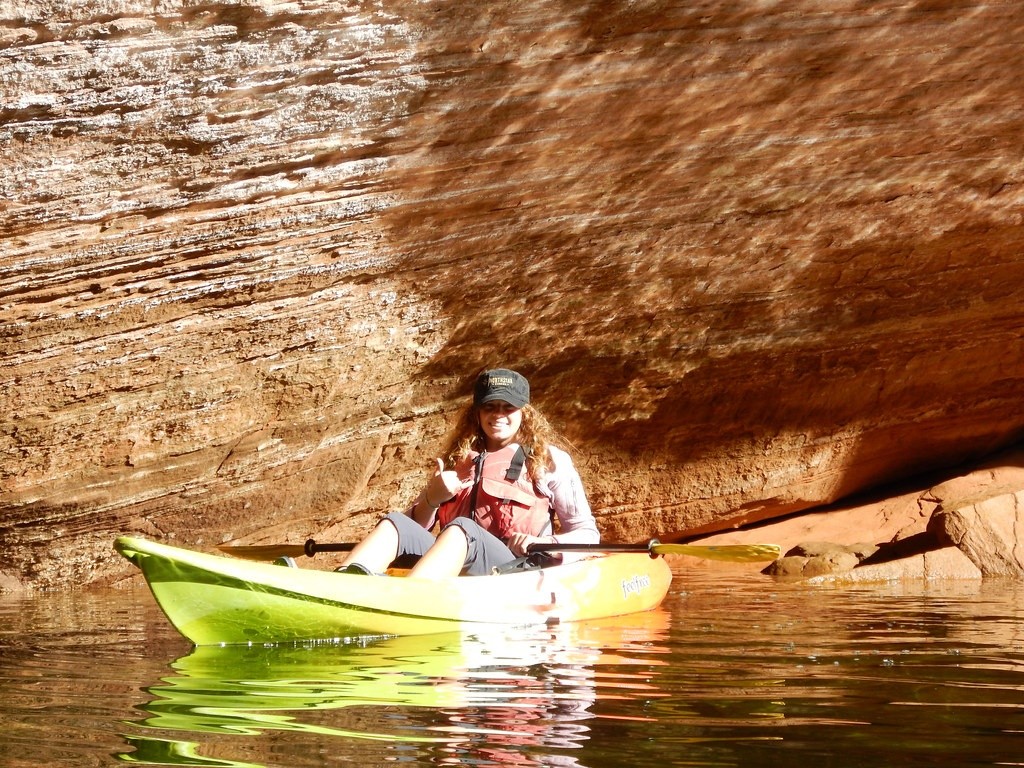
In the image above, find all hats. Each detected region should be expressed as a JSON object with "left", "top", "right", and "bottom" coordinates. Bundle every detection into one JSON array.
[{"left": 474, "top": 367, "right": 530, "bottom": 408}]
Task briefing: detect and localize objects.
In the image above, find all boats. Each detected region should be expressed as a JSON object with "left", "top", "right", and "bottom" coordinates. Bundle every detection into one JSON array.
[{"left": 110, "top": 537, "right": 672, "bottom": 646}]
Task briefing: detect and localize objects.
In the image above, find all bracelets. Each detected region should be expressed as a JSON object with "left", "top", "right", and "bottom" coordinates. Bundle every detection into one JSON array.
[{"left": 424, "top": 492, "right": 440, "bottom": 509}]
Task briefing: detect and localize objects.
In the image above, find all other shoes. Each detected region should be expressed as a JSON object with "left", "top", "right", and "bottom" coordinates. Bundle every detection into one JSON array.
[
  {"left": 274, "top": 556, "right": 291, "bottom": 567},
  {"left": 348, "top": 562, "right": 372, "bottom": 575}
]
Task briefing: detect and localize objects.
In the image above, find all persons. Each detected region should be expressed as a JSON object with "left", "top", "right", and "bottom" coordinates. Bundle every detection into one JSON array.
[{"left": 271, "top": 368, "right": 600, "bottom": 577}]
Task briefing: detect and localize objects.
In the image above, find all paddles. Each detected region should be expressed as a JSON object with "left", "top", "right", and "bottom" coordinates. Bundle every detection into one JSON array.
[{"left": 220, "top": 531, "right": 783, "bottom": 563}]
